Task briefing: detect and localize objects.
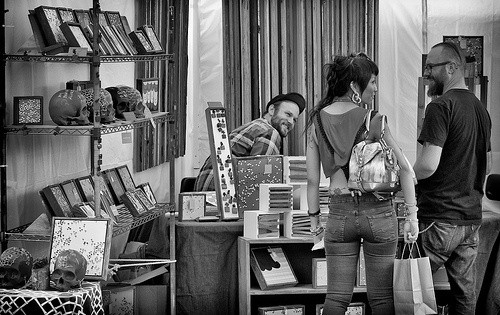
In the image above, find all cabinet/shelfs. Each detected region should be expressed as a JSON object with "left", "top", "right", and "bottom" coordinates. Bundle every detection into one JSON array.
[
  {"left": 237, "top": 236, "right": 368, "bottom": 315},
  {"left": 1, "top": 2, "right": 179, "bottom": 315}
]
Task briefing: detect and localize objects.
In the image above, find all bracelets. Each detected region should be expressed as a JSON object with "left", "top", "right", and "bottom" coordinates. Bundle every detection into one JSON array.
[
  {"left": 404, "top": 218, "right": 418, "bottom": 223},
  {"left": 405, "top": 201, "right": 416, "bottom": 207},
  {"left": 308, "top": 209, "right": 321, "bottom": 217},
  {"left": 310, "top": 224, "right": 324, "bottom": 237},
  {"left": 405, "top": 207, "right": 418, "bottom": 215}
]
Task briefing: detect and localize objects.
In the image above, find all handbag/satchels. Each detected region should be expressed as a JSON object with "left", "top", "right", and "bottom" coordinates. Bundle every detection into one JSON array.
[
  {"left": 393, "top": 240, "right": 438, "bottom": 315},
  {"left": 347, "top": 140, "right": 419, "bottom": 193}
]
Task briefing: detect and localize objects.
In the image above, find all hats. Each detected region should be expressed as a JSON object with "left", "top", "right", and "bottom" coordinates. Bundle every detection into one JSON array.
[{"left": 267, "top": 91, "right": 306, "bottom": 116}]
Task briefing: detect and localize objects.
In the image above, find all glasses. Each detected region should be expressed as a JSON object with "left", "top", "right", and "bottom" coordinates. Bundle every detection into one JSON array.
[
  {"left": 342, "top": 50, "right": 373, "bottom": 71},
  {"left": 423, "top": 60, "right": 454, "bottom": 71},
  {"left": 431, "top": 41, "right": 462, "bottom": 61}
]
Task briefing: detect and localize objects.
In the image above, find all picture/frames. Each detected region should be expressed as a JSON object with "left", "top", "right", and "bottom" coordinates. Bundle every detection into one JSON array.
[
  {"left": 179, "top": 193, "right": 207, "bottom": 223},
  {"left": 315, "top": 303, "right": 366, "bottom": 315},
  {"left": 136, "top": 75, "right": 160, "bottom": 110},
  {"left": 311, "top": 258, "right": 329, "bottom": 289},
  {"left": 260, "top": 306, "right": 306, "bottom": 315},
  {"left": 49, "top": 217, "right": 111, "bottom": 283},
  {"left": 41, "top": 164, "right": 157, "bottom": 226},
  {"left": 13, "top": 94, "right": 44, "bottom": 127},
  {"left": 249, "top": 247, "right": 299, "bottom": 290},
  {"left": 355, "top": 243, "right": 368, "bottom": 289},
  {"left": 34, "top": 4, "right": 165, "bottom": 59}
]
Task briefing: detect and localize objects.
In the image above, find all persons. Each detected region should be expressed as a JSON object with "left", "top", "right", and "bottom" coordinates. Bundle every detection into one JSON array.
[
  {"left": 300, "top": 51, "right": 420, "bottom": 315},
  {"left": 400, "top": 41, "right": 492, "bottom": 315},
  {"left": 194, "top": 92, "right": 306, "bottom": 191}
]
